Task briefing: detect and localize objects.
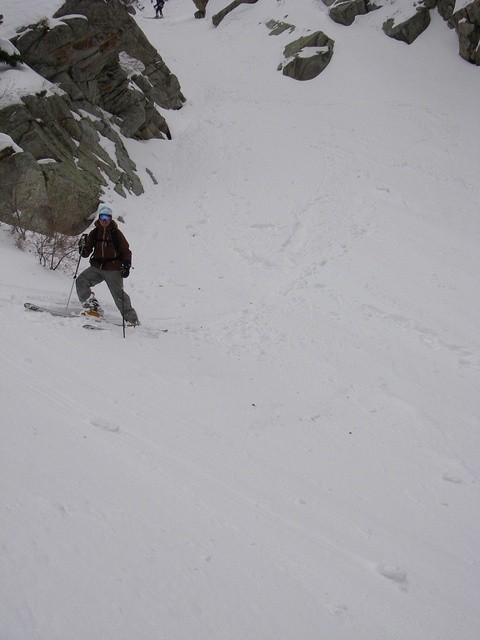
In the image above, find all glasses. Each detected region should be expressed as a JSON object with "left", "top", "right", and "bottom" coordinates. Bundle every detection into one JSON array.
[{"left": 99, "top": 214, "right": 112, "bottom": 222}]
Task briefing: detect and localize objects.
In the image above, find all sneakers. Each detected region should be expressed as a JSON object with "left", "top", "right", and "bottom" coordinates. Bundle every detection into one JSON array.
[
  {"left": 82, "top": 292, "right": 104, "bottom": 313},
  {"left": 125, "top": 320, "right": 140, "bottom": 327}
]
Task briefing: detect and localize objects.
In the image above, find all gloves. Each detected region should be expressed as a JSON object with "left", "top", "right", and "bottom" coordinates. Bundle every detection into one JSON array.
[
  {"left": 78, "top": 234, "right": 88, "bottom": 247},
  {"left": 121, "top": 261, "right": 131, "bottom": 278}
]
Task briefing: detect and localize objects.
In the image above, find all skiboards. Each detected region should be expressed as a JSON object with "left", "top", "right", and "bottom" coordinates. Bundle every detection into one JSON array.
[{"left": 23, "top": 303, "right": 169, "bottom": 332}]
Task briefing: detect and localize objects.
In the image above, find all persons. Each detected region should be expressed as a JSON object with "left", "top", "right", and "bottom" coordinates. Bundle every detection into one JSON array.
[{"left": 73, "top": 205, "right": 140, "bottom": 327}]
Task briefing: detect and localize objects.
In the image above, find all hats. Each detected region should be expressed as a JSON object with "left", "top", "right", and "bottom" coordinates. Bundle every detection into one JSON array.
[{"left": 99, "top": 206, "right": 113, "bottom": 217}]
